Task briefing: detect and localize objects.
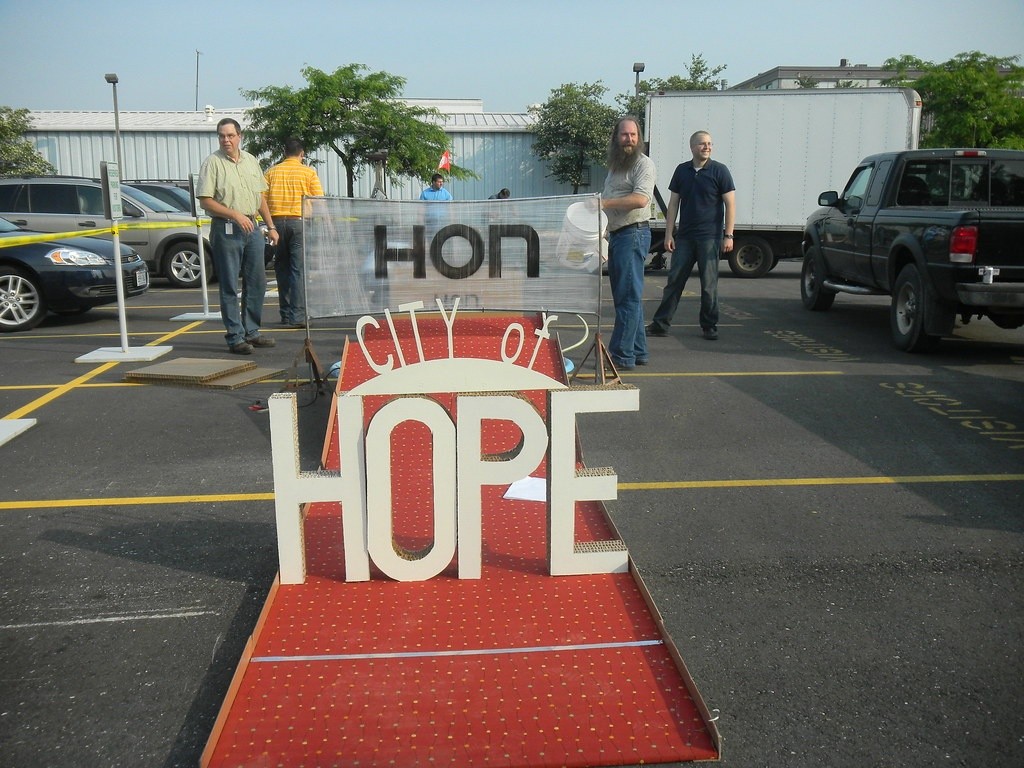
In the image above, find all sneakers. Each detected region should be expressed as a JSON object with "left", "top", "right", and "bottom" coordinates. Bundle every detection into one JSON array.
[{"left": 230, "top": 336, "right": 276, "bottom": 354}]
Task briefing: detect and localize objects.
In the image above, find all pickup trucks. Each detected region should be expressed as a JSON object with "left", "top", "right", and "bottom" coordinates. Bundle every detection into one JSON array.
[{"left": 800, "top": 147, "right": 1023, "bottom": 356}]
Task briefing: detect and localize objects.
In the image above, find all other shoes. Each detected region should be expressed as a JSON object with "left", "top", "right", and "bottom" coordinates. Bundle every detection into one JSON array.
[
  {"left": 282, "top": 314, "right": 312, "bottom": 327},
  {"left": 703, "top": 325, "right": 720, "bottom": 340},
  {"left": 645, "top": 323, "right": 670, "bottom": 337},
  {"left": 595, "top": 359, "right": 647, "bottom": 371}
]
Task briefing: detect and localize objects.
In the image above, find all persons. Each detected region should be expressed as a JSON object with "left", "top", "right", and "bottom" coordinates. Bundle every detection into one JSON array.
[
  {"left": 196, "top": 118, "right": 279, "bottom": 354},
  {"left": 586, "top": 116, "right": 656, "bottom": 372},
  {"left": 645, "top": 130, "right": 736, "bottom": 341},
  {"left": 480, "top": 189, "right": 518, "bottom": 263},
  {"left": 264, "top": 140, "right": 334, "bottom": 327},
  {"left": 419, "top": 173, "right": 453, "bottom": 266}
]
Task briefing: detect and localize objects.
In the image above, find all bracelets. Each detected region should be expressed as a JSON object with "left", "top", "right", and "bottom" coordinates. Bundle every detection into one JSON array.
[{"left": 267, "top": 225, "right": 277, "bottom": 230}]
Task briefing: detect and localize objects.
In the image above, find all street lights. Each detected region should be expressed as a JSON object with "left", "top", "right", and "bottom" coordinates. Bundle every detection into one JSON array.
[
  {"left": 633, "top": 63, "right": 645, "bottom": 120},
  {"left": 104, "top": 73, "right": 123, "bottom": 183}
]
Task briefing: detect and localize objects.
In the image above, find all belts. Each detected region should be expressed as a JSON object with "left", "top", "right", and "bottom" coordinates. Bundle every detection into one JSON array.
[{"left": 610, "top": 221, "right": 648, "bottom": 236}]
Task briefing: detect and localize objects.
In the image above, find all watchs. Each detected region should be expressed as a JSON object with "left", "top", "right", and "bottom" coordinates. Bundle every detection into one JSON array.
[{"left": 725, "top": 233, "right": 735, "bottom": 239}]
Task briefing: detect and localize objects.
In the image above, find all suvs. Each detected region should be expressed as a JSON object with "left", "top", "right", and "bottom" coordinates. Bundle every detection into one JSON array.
[
  {"left": 120, "top": 177, "right": 192, "bottom": 192},
  {"left": 0, "top": 174, "right": 220, "bottom": 288}
]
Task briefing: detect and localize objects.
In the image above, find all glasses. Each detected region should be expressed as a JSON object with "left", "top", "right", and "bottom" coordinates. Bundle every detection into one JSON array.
[{"left": 218, "top": 132, "right": 239, "bottom": 139}]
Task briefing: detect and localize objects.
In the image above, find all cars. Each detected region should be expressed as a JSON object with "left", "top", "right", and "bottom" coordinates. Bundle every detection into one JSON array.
[
  {"left": 122, "top": 182, "right": 274, "bottom": 277},
  {"left": 0, "top": 218, "right": 152, "bottom": 334}
]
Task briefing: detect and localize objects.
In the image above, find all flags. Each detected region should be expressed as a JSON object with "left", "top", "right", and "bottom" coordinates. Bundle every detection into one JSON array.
[{"left": 438, "top": 151, "right": 450, "bottom": 173}]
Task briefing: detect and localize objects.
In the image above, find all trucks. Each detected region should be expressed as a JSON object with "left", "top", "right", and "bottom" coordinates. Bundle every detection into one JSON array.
[{"left": 647, "top": 85, "right": 980, "bottom": 278}]
